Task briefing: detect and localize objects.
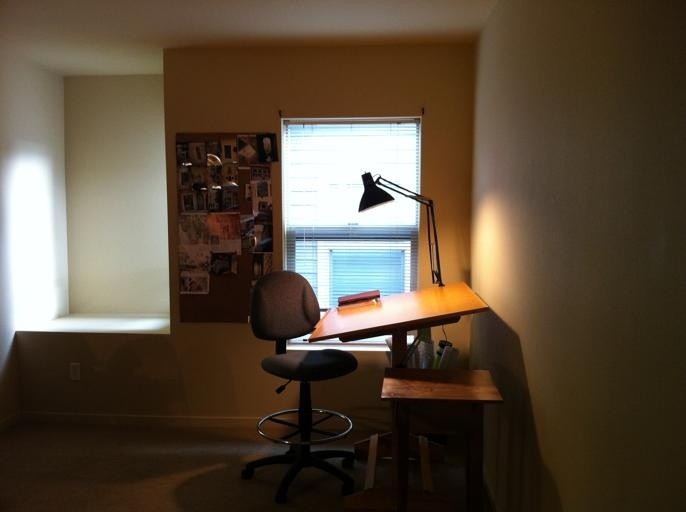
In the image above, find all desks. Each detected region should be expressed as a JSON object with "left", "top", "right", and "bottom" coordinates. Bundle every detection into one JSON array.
[{"left": 307, "top": 280, "right": 505, "bottom": 511}]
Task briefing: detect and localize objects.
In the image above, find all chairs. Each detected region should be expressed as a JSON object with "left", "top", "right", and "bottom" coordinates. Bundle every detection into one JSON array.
[{"left": 240, "top": 268, "right": 358, "bottom": 505}]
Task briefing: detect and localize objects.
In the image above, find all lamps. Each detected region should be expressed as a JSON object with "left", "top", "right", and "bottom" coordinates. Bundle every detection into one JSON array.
[{"left": 356, "top": 170, "right": 444, "bottom": 287}]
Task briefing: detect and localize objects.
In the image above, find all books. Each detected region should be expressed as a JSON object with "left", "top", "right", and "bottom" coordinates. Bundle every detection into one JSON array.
[
  {"left": 338, "top": 288, "right": 381, "bottom": 306},
  {"left": 336, "top": 299, "right": 379, "bottom": 310}
]
